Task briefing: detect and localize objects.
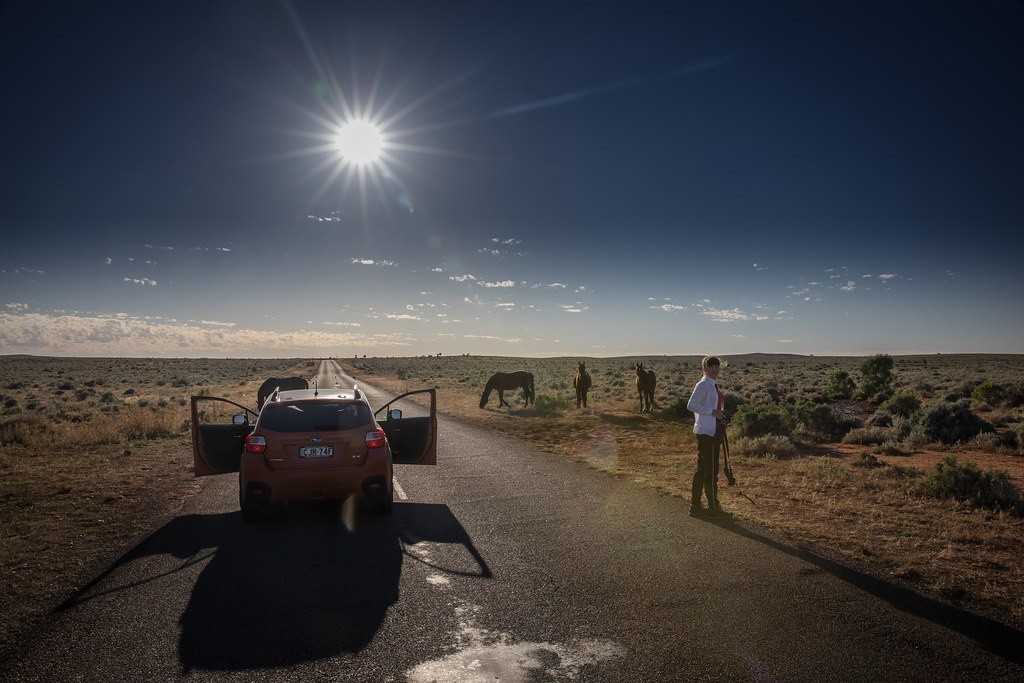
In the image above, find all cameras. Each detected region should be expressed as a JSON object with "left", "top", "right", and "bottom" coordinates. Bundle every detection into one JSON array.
[{"left": 718, "top": 411, "right": 732, "bottom": 427}]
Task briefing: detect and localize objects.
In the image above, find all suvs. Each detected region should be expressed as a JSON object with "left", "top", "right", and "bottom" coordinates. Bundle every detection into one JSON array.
[{"left": 189, "top": 384, "right": 439, "bottom": 526}]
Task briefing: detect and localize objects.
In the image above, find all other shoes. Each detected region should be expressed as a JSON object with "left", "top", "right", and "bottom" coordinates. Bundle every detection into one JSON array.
[
  {"left": 689, "top": 501, "right": 708, "bottom": 517},
  {"left": 706, "top": 501, "right": 733, "bottom": 520}
]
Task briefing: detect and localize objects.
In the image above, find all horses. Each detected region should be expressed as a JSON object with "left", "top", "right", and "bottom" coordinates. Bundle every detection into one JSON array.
[
  {"left": 479, "top": 370, "right": 535, "bottom": 408},
  {"left": 573, "top": 361, "right": 592, "bottom": 409},
  {"left": 635, "top": 362, "right": 656, "bottom": 412}
]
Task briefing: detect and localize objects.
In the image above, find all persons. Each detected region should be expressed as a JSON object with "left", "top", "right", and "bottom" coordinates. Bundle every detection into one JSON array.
[{"left": 687, "top": 355, "right": 734, "bottom": 520}]
